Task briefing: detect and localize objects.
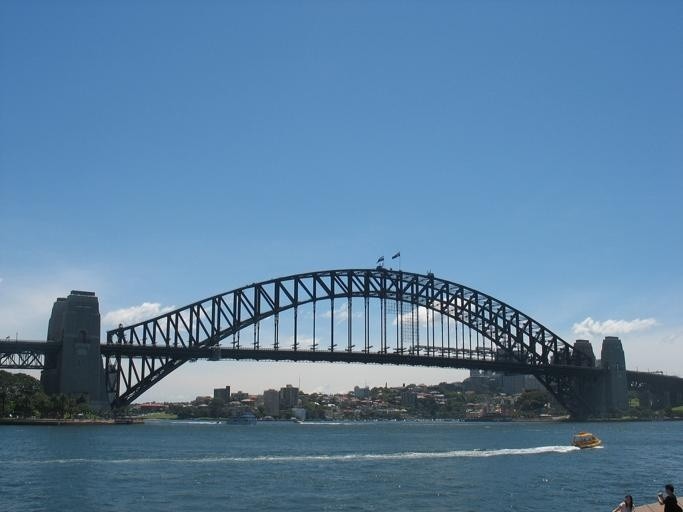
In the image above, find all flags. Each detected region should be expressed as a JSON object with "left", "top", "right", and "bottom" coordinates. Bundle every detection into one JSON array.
[
  {"left": 392, "top": 251, "right": 400, "bottom": 259},
  {"left": 376, "top": 255, "right": 384, "bottom": 263}
]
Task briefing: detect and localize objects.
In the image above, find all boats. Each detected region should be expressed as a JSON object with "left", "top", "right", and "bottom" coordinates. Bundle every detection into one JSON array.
[{"left": 570, "top": 433, "right": 602, "bottom": 449}]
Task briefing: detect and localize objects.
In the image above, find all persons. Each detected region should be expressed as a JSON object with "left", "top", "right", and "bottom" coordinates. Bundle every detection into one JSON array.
[
  {"left": 657, "top": 484, "right": 677, "bottom": 512},
  {"left": 612, "top": 495, "right": 635, "bottom": 512}
]
